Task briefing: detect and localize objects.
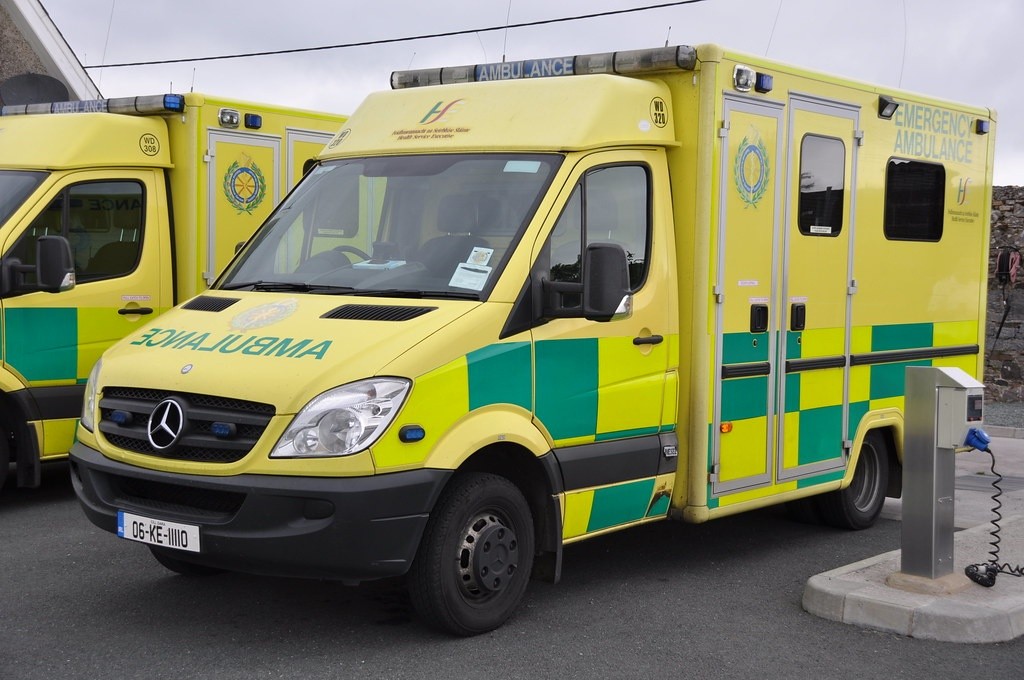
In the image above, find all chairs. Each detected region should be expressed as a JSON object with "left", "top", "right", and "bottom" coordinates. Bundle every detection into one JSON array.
[
  {"left": 17, "top": 211, "right": 50, "bottom": 265},
  {"left": 411, "top": 194, "right": 496, "bottom": 275},
  {"left": 553, "top": 190, "right": 631, "bottom": 264},
  {"left": 84, "top": 199, "right": 139, "bottom": 276}
]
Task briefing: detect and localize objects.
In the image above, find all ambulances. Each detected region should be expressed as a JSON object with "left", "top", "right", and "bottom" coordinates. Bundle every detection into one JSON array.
[
  {"left": 70, "top": 43, "right": 990, "bottom": 639},
  {"left": 0, "top": 92, "right": 445, "bottom": 514}
]
